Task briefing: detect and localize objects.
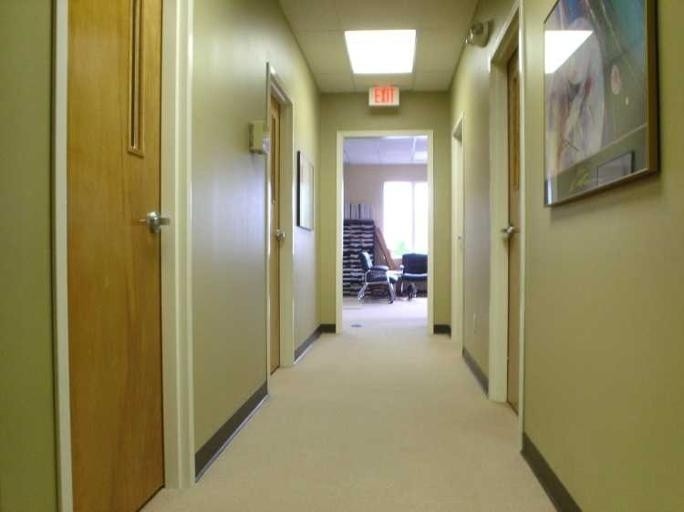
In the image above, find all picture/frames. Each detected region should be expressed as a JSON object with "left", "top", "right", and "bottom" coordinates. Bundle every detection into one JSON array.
[{"left": 541, "top": 0, "right": 660, "bottom": 208}]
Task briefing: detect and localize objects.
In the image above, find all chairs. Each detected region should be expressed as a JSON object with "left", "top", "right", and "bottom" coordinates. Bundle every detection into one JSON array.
[
  {"left": 398, "top": 254, "right": 427, "bottom": 300},
  {"left": 357, "top": 251, "right": 398, "bottom": 303}
]
